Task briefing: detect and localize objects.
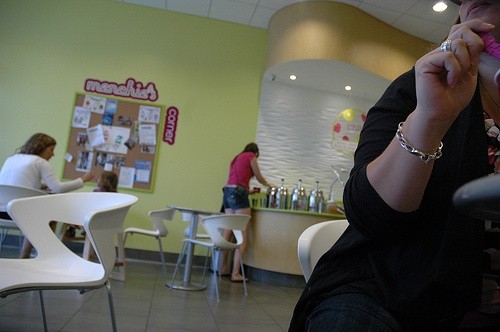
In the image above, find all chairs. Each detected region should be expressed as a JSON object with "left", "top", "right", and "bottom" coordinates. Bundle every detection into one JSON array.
[
  {"left": 297, "top": 218, "right": 350, "bottom": 283},
  {"left": 123, "top": 208, "right": 252, "bottom": 303},
  {"left": 0, "top": 184, "right": 140, "bottom": 332}
]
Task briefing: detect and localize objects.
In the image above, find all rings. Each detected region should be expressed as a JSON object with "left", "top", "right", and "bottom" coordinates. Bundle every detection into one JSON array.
[{"left": 441, "top": 40, "right": 451, "bottom": 51}]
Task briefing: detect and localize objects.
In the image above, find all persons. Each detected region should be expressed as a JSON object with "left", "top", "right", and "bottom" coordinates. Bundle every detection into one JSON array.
[
  {"left": 219, "top": 143, "right": 271, "bottom": 282},
  {"left": 0, "top": 133, "right": 95, "bottom": 220},
  {"left": 289, "top": 0, "right": 500, "bottom": 332},
  {"left": 65, "top": 170, "right": 118, "bottom": 237}
]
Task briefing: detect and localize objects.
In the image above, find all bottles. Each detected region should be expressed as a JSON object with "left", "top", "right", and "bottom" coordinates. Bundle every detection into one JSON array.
[{"left": 249, "top": 186, "right": 324, "bottom": 213}]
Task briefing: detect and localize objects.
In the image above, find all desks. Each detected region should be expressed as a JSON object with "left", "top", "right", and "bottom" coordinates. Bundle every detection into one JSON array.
[{"left": 165, "top": 203, "right": 223, "bottom": 291}]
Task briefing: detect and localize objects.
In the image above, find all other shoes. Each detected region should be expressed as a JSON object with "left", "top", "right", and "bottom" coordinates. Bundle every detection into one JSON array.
[
  {"left": 218, "top": 273, "right": 231, "bottom": 277},
  {"left": 232, "top": 278, "right": 249, "bottom": 282}
]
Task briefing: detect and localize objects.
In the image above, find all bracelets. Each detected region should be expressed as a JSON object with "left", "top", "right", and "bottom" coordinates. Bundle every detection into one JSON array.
[{"left": 396, "top": 123, "right": 443, "bottom": 163}]
